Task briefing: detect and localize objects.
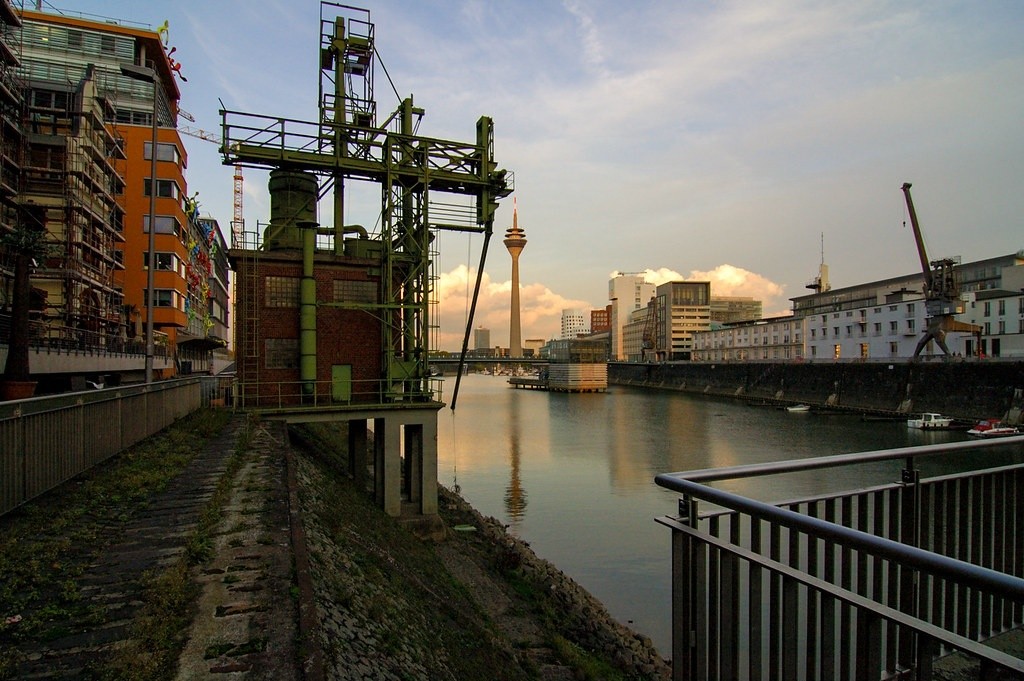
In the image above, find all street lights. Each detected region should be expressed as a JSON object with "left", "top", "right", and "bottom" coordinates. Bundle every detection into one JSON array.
[{"left": 120, "top": 63, "right": 160, "bottom": 383}]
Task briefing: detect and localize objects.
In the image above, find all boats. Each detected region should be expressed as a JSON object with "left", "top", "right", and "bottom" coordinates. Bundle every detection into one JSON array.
[
  {"left": 966, "top": 419, "right": 1021, "bottom": 437},
  {"left": 482, "top": 364, "right": 539, "bottom": 378},
  {"left": 787, "top": 403, "right": 812, "bottom": 412},
  {"left": 907, "top": 412, "right": 954, "bottom": 430}
]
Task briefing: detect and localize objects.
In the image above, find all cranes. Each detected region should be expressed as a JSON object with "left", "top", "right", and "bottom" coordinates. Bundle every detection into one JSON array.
[
  {"left": 898, "top": 182, "right": 994, "bottom": 362},
  {"left": 176, "top": 124, "right": 244, "bottom": 365}
]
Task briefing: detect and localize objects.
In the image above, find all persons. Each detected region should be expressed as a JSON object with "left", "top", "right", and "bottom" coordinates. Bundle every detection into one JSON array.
[
  {"left": 952, "top": 352, "right": 955, "bottom": 359},
  {"left": 958, "top": 352, "right": 961, "bottom": 357},
  {"left": 209, "top": 364, "right": 213, "bottom": 376},
  {"left": 981, "top": 353, "right": 985, "bottom": 360}
]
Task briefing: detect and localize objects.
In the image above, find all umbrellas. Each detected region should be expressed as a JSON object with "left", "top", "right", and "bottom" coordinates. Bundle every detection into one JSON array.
[
  {"left": 119, "top": 312, "right": 127, "bottom": 352},
  {"left": 133, "top": 309, "right": 143, "bottom": 354}
]
